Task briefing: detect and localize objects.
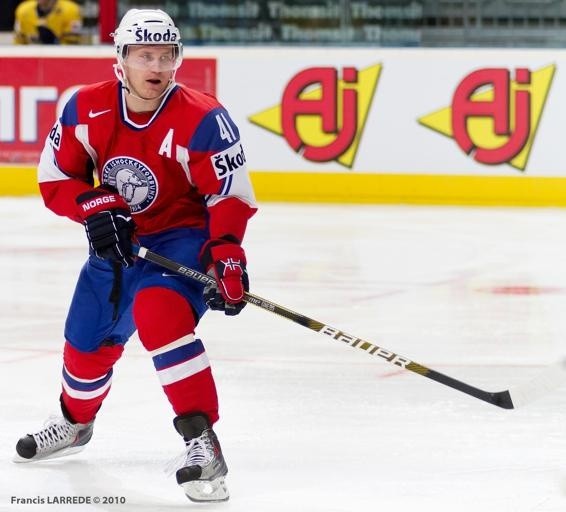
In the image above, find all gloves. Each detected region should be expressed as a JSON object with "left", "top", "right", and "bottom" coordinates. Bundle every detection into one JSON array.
[
  {"left": 76, "top": 184, "right": 136, "bottom": 267},
  {"left": 196, "top": 239, "right": 249, "bottom": 316}
]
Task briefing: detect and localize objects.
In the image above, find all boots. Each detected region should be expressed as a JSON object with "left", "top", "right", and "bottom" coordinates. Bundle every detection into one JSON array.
[
  {"left": 16, "top": 394, "right": 102, "bottom": 460},
  {"left": 173, "top": 415, "right": 228, "bottom": 485}
]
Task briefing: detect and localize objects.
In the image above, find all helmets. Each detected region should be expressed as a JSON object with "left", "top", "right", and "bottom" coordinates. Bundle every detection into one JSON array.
[{"left": 109, "top": 8, "right": 184, "bottom": 73}]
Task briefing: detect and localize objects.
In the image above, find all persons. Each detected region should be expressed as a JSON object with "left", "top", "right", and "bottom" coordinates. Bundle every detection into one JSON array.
[
  {"left": 12, "top": 1, "right": 93, "bottom": 48},
  {"left": 11, "top": 4, "right": 259, "bottom": 486}
]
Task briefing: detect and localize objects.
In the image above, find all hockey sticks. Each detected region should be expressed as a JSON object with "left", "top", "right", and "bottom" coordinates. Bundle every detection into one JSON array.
[{"left": 132, "top": 243, "right": 566, "bottom": 410}]
